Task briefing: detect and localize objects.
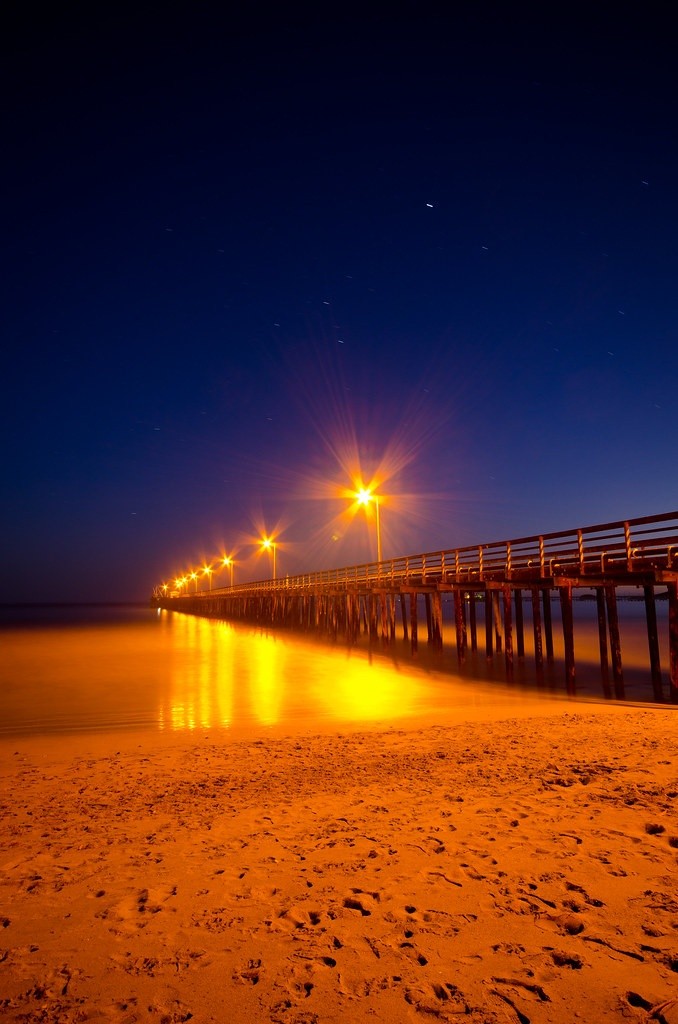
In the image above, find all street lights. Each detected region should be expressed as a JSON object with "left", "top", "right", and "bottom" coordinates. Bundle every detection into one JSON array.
[{"left": 161, "top": 490, "right": 382, "bottom": 598}]
